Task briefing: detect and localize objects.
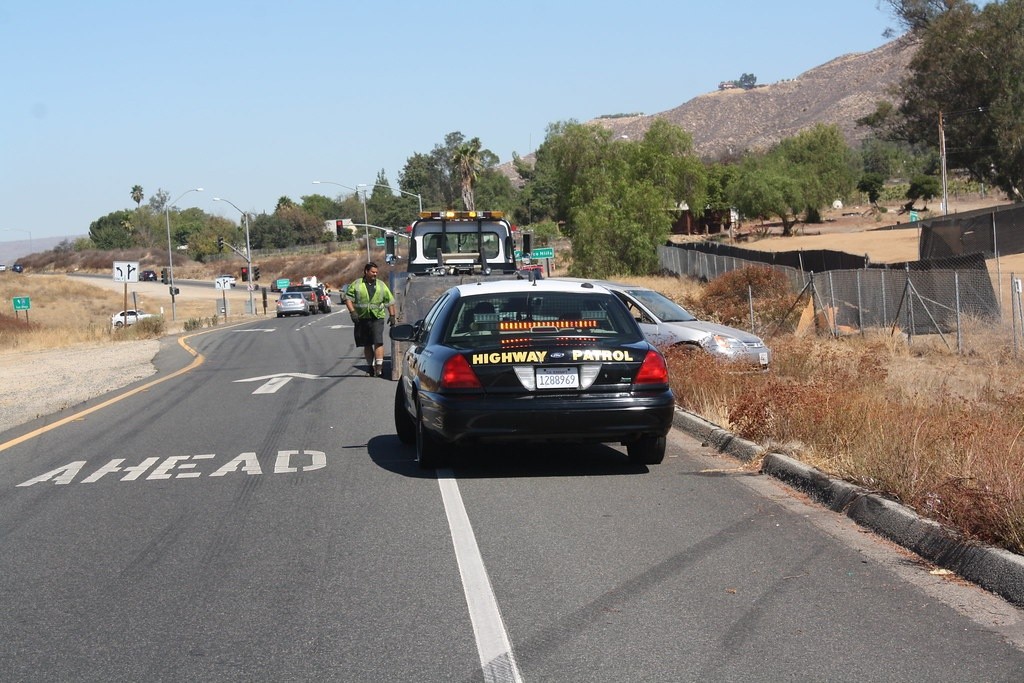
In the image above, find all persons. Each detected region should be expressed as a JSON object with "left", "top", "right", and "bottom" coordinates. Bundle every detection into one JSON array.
[{"left": 342, "top": 262, "right": 395, "bottom": 377}]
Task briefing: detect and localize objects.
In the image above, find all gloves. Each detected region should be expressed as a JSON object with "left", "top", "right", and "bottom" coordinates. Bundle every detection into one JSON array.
[
  {"left": 387, "top": 315, "right": 396, "bottom": 326},
  {"left": 351, "top": 314, "right": 359, "bottom": 323}
]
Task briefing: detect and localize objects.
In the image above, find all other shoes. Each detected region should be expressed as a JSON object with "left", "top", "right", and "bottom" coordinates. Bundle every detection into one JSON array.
[
  {"left": 365, "top": 371, "right": 374, "bottom": 376},
  {"left": 376, "top": 370, "right": 383, "bottom": 378}
]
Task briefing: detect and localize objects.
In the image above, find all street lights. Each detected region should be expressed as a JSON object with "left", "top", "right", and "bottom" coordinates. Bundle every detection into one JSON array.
[
  {"left": 311, "top": 181, "right": 370, "bottom": 265},
  {"left": 4, "top": 228, "right": 33, "bottom": 267},
  {"left": 358, "top": 184, "right": 422, "bottom": 212},
  {"left": 212, "top": 198, "right": 255, "bottom": 315},
  {"left": 166, "top": 188, "right": 204, "bottom": 322}
]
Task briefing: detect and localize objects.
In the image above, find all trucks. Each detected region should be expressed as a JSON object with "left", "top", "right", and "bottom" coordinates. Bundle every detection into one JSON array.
[{"left": 390, "top": 211, "right": 537, "bottom": 381}]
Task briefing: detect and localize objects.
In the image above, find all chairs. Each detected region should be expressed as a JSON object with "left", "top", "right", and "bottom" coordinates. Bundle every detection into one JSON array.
[
  {"left": 473, "top": 302, "right": 495, "bottom": 313},
  {"left": 560, "top": 313, "right": 584, "bottom": 320},
  {"left": 426, "top": 235, "right": 451, "bottom": 259}
]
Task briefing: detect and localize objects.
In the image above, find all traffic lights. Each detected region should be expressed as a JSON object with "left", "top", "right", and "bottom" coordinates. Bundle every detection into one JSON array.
[
  {"left": 385, "top": 235, "right": 395, "bottom": 264},
  {"left": 241, "top": 267, "right": 248, "bottom": 282},
  {"left": 161, "top": 268, "right": 168, "bottom": 284},
  {"left": 253, "top": 266, "right": 260, "bottom": 281},
  {"left": 336, "top": 220, "right": 344, "bottom": 235},
  {"left": 173, "top": 288, "right": 180, "bottom": 295},
  {"left": 218, "top": 236, "right": 224, "bottom": 252}
]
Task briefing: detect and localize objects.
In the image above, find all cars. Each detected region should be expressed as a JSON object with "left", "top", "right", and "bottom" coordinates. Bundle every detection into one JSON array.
[
  {"left": 112, "top": 309, "right": 156, "bottom": 329},
  {"left": 0, "top": 264, "right": 6, "bottom": 272},
  {"left": 541, "top": 277, "right": 773, "bottom": 376},
  {"left": 339, "top": 283, "right": 351, "bottom": 304},
  {"left": 139, "top": 270, "right": 158, "bottom": 282},
  {"left": 12, "top": 264, "right": 24, "bottom": 274},
  {"left": 275, "top": 291, "right": 310, "bottom": 318},
  {"left": 270, "top": 276, "right": 332, "bottom": 315},
  {"left": 389, "top": 278, "right": 676, "bottom": 472}
]
